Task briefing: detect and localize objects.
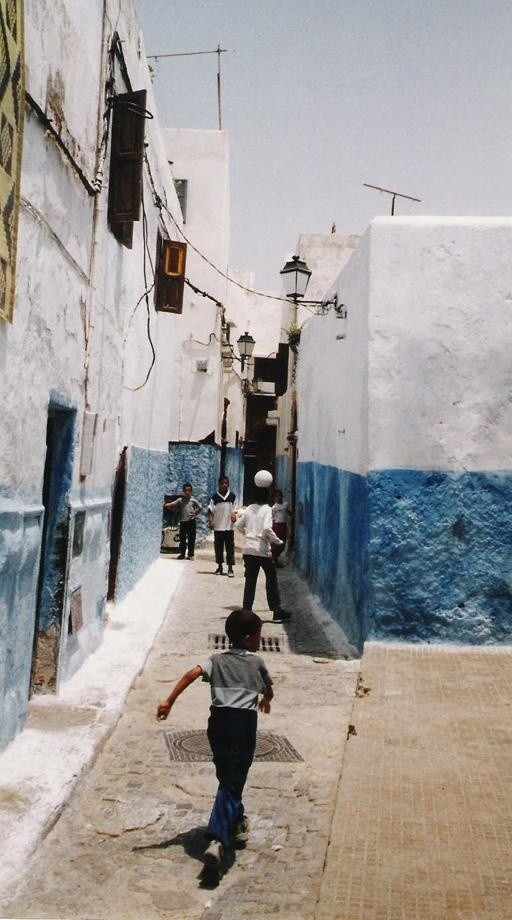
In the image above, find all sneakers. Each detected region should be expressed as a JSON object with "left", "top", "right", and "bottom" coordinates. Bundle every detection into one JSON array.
[
  {"left": 187, "top": 556, "right": 194, "bottom": 560},
  {"left": 228, "top": 569, "right": 234, "bottom": 577},
  {"left": 215, "top": 568, "right": 223, "bottom": 575},
  {"left": 273, "top": 610, "right": 292, "bottom": 622},
  {"left": 178, "top": 555, "right": 185, "bottom": 559},
  {"left": 202, "top": 839, "right": 224, "bottom": 883},
  {"left": 235, "top": 815, "right": 249, "bottom": 843}
]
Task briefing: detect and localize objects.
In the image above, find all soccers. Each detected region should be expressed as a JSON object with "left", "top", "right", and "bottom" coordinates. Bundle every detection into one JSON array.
[{"left": 255, "top": 470, "right": 273, "bottom": 487}]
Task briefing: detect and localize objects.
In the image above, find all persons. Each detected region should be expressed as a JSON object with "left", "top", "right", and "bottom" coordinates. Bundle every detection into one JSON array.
[
  {"left": 156, "top": 610, "right": 274, "bottom": 888},
  {"left": 234, "top": 487, "right": 293, "bottom": 624},
  {"left": 164, "top": 482, "right": 203, "bottom": 560},
  {"left": 270, "top": 490, "right": 292, "bottom": 568},
  {"left": 207, "top": 476, "right": 238, "bottom": 577}
]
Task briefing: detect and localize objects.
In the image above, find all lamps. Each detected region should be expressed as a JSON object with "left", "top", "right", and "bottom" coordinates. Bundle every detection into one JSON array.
[
  {"left": 276, "top": 251, "right": 351, "bottom": 322},
  {"left": 221, "top": 327, "right": 257, "bottom": 375}
]
